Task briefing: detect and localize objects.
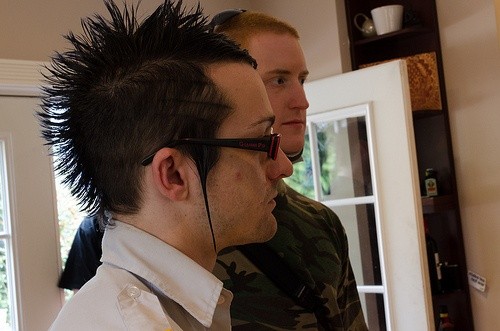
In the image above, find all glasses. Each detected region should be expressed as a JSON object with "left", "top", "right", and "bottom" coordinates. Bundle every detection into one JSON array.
[
  {"left": 141, "top": 125, "right": 282, "bottom": 167},
  {"left": 208, "top": 8, "right": 246, "bottom": 34}
]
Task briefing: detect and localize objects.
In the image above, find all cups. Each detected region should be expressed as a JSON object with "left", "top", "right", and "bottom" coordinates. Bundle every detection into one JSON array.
[{"left": 371, "top": 6, "right": 403, "bottom": 36}]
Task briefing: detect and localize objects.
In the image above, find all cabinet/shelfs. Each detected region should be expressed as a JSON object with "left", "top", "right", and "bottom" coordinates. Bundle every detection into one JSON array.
[{"left": 340, "top": 0, "right": 476, "bottom": 331}]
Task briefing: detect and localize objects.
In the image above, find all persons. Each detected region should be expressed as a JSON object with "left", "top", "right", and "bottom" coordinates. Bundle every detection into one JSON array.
[
  {"left": 57, "top": 212, "right": 108, "bottom": 290},
  {"left": 38, "top": 0, "right": 293, "bottom": 331},
  {"left": 207, "top": 10, "right": 368, "bottom": 331}
]
